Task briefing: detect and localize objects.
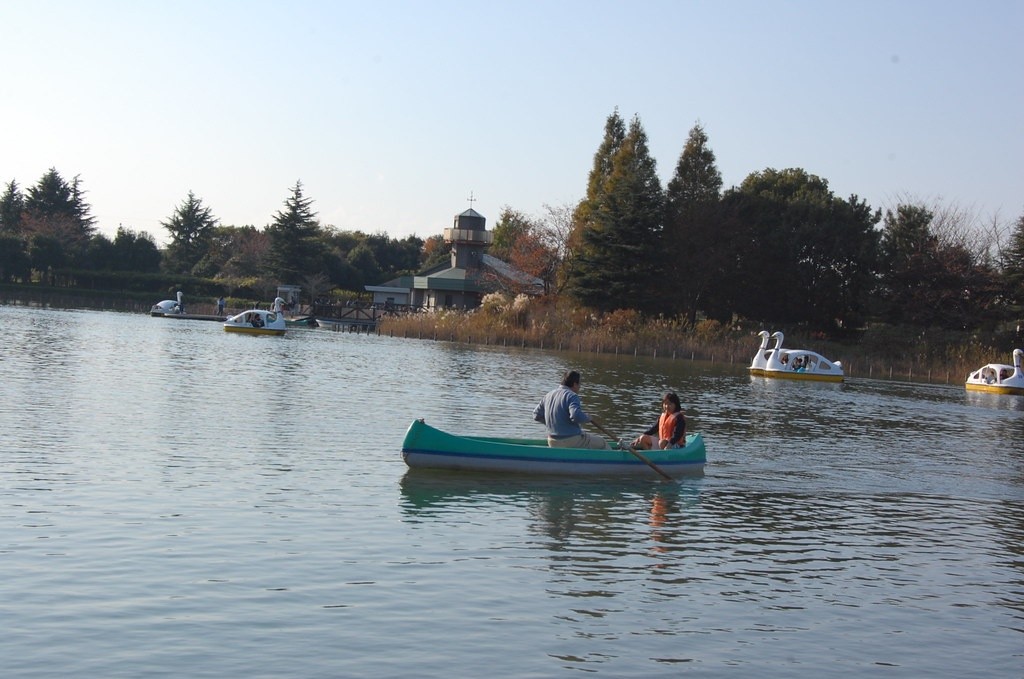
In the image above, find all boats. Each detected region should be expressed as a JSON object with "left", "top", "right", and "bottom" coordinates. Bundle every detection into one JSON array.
[
  {"left": 150, "top": 291, "right": 184, "bottom": 317},
  {"left": 400, "top": 416, "right": 708, "bottom": 476},
  {"left": 223, "top": 295, "right": 288, "bottom": 337},
  {"left": 745, "top": 330, "right": 844, "bottom": 383},
  {"left": 965, "top": 348, "right": 1024, "bottom": 396}
]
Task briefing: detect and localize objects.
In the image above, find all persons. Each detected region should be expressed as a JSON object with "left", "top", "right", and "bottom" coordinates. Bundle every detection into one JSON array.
[
  {"left": 533, "top": 370, "right": 612, "bottom": 450},
  {"left": 255, "top": 300, "right": 261, "bottom": 309},
  {"left": 630, "top": 393, "right": 685, "bottom": 449},
  {"left": 217, "top": 296, "right": 225, "bottom": 316},
  {"left": 370, "top": 303, "right": 377, "bottom": 310},
  {"left": 251, "top": 313, "right": 265, "bottom": 327},
  {"left": 315, "top": 297, "right": 355, "bottom": 307},
  {"left": 988, "top": 370, "right": 1008, "bottom": 383},
  {"left": 781, "top": 354, "right": 808, "bottom": 371},
  {"left": 180, "top": 302, "right": 184, "bottom": 314},
  {"left": 290, "top": 296, "right": 297, "bottom": 318}
]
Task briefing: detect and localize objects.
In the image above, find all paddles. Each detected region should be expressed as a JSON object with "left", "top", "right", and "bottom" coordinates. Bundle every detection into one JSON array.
[{"left": 590, "top": 419, "right": 675, "bottom": 479}]
